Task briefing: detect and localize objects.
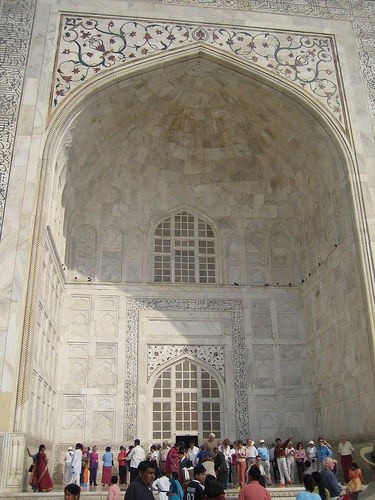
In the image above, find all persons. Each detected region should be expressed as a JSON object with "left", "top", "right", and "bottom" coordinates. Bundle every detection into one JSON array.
[
  {"left": 182, "top": 464, "right": 206, "bottom": 500},
  {"left": 124, "top": 460, "right": 155, "bottom": 500},
  {"left": 64, "top": 484, "right": 80, "bottom": 500},
  {"left": 27, "top": 444, "right": 53, "bottom": 492},
  {"left": 108, "top": 476, "right": 120, "bottom": 500},
  {"left": 65, "top": 433, "right": 375, "bottom": 500},
  {"left": 152, "top": 470, "right": 171, "bottom": 500}
]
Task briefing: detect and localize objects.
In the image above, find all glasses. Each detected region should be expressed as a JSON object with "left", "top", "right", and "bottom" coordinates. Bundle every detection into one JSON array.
[
  {"left": 319, "top": 441, "right": 323, "bottom": 442},
  {"left": 68, "top": 450, "right": 72, "bottom": 451}
]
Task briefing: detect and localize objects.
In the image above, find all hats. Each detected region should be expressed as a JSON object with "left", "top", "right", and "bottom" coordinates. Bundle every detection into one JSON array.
[
  {"left": 308, "top": 441, "right": 314, "bottom": 444},
  {"left": 259, "top": 440, "right": 264, "bottom": 443},
  {"left": 360, "top": 444, "right": 375, "bottom": 465},
  {"left": 209, "top": 433, "right": 214, "bottom": 438},
  {"left": 68, "top": 447, "right": 72, "bottom": 450}
]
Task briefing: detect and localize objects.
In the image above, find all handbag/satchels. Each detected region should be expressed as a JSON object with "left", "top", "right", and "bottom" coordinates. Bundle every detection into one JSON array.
[{"left": 112, "top": 454, "right": 113, "bottom": 465}]
